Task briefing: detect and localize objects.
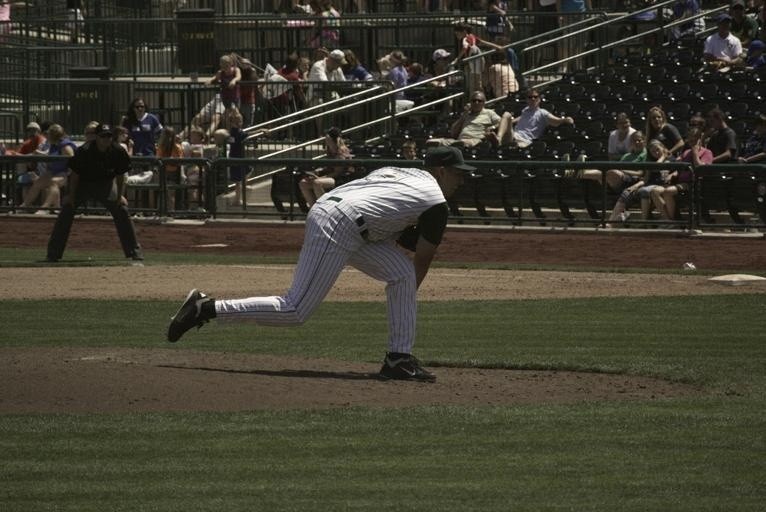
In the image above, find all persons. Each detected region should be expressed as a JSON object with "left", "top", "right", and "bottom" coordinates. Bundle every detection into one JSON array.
[
  {"left": 0, "top": 1, "right": 765, "bottom": 260},
  {"left": 167, "top": 147, "right": 479, "bottom": 381}
]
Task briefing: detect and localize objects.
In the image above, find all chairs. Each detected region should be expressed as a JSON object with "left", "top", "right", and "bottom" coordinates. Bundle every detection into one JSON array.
[{"left": 270, "top": 0, "right": 766, "bottom": 229}]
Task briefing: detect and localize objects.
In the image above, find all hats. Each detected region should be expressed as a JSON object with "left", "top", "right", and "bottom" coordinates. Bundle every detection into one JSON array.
[
  {"left": 424, "top": 146, "right": 477, "bottom": 174},
  {"left": 95, "top": 123, "right": 112, "bottom": 138},
  {"left": 431, "top": 48, "right": 449, "bottom": 61},
  {"left": 731, "top": 0, "right": 744, "bottom": 9},
  {"left": 330, "top": 49, "right": 348, "bottom": 66},
  {"left": 323, "top": 126, "right": 342, "bottom": 141},
  {"left": 716, "top": 14, "right": 731, "bottom": 24}
]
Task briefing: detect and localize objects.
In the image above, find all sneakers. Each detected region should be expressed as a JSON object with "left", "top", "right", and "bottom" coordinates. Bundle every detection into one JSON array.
[
  {"left": 377, "top": 351, "right": 436, "bottom": 383},
  {"left": 167, "top": 288, "right": 213, "bottom": 343}
]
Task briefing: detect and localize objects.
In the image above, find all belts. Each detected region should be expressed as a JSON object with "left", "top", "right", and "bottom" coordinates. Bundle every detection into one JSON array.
[{"left": 327, "top": 196, "right": 368, "bottom": 242}]
